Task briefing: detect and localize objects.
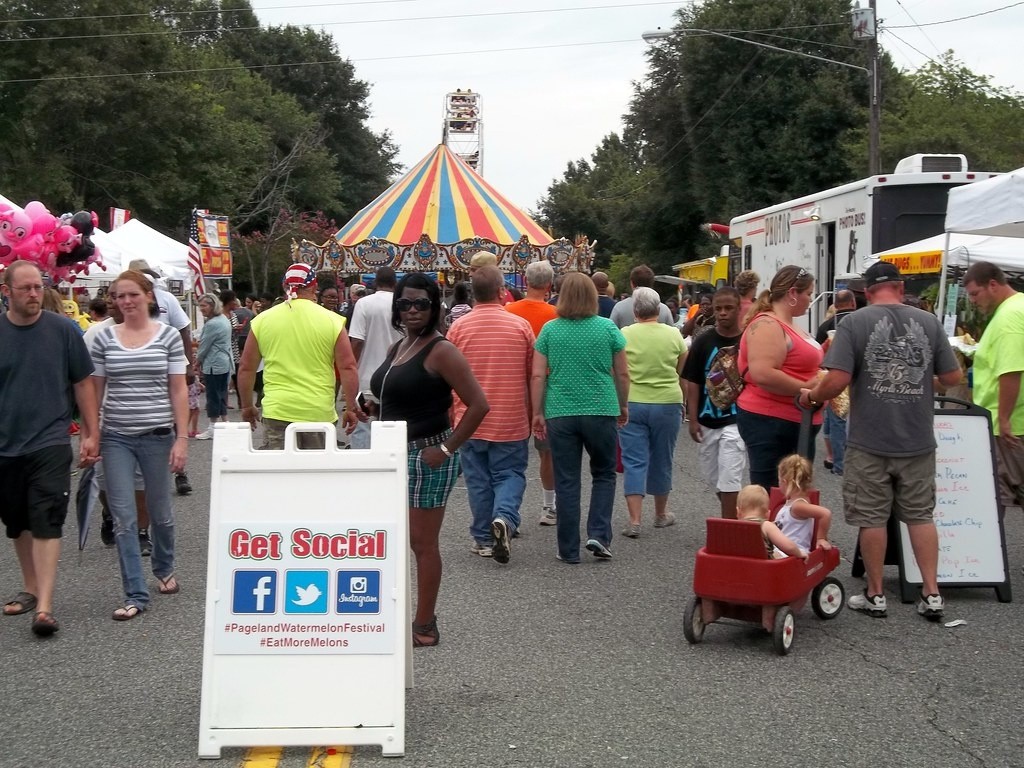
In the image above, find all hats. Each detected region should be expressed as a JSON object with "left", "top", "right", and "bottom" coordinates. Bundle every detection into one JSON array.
[
  {"left": 470, "top": 250, "right": 497, "bottom": 266},
  {"left": 863, "top": 261, "right": 902, "bottom": 288},
  {"left": 129, "top": 259, "right": 161, "bottom": 279}
]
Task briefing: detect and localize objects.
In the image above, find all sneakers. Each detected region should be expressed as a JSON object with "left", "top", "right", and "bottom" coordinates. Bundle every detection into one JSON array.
[
  {"left": 912, "top": 590, "right": 944, "bottom": 618},
  {"left": 194, "top": 428, "right": 214, "bottom": 440},
  {"left": 848, "top": 588, "right": 888, "bottom": 617},
  {"left": 175, "top": 470, "right": 191, "bottom": 493}
]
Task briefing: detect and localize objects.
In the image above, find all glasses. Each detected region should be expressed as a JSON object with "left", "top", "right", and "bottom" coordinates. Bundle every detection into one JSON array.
[
  {"left": 7, "top": 285, "right": 46, "bottom": 294},
  {"left": 323, "top": 295, "right": 339, "bottom": 300},
  {"left": 395, "top": 297, "right": 432, "bottom": 312}
]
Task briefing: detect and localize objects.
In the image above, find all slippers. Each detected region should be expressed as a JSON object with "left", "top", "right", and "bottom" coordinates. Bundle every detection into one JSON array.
[
  {"left": 111, "top": 604, "right": 142, "bottom": 620},
  {"left": 159, "top": 574, "right": 179, "bottom": 594},
  {"left": 3, "top": 591, "right": 58, "bottom": 635}
]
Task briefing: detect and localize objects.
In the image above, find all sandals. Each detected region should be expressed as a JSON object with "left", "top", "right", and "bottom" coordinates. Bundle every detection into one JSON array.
[{"left": 412, "top": 615, "right": 440, "bottom": 647}]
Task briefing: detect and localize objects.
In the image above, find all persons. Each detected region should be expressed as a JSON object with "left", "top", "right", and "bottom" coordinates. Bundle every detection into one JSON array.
[
  {"left": 725, "top": 485, "right": 810, "bottom": 611},
  {"left": 529, "top": 272, "right": 631, "bottom": 565},
  {"left": 800, "top": 260, "right": 965, "bottom": 618},
  {"left": 0, "top": 260, "right": 101, "bottom": 636},
  {"left": 736, "top": 264, "right": 831, "bottom": 495},
  {"left": 772, "top": 454, "right": 832, "bottom": 560},
  {"left": 815, "top": 290, "right": 858, "bottom": 475},
  {"left": 619, "top": 286, "right": 689, "bottom": 537},
  {"left": 961, "top": 260, "right": 1024, "bottom": 518},
  {"left": 445, "top": 264, "right": 536, "bottom": 564},
  {"left": 353, "top": 271, "right": 490, "bottom": 648},
  {"left": 0, "top": 251, "right": 762, "bottom": 556},
  {"left": 236, "top": 263, "right": 361, "bottom": 448},
  {"left": 78, "top": 271, "right": 190, "bottom": 621},
  {"left": 681, "top": 286, "right": 748, "bottom": 522}
]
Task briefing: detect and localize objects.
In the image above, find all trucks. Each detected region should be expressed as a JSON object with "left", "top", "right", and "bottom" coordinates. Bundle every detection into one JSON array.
[{"left": 727, "top": 152, "right": 1014, "bottom": 382}]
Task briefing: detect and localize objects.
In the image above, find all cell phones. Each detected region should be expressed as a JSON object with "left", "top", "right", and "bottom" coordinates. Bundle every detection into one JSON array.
[{"left": 355, "top": 391, "right": 370, "bottom": 417}]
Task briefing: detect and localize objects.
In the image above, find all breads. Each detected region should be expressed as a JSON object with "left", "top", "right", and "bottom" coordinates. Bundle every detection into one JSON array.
[{"left": 963, "top": 332, "right": 975, "bottom": 345}]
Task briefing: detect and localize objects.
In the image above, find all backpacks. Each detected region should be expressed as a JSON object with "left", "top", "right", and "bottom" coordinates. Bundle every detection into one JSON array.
[{"left": 706, "top": 314, "right": 771, "bottom": 409}]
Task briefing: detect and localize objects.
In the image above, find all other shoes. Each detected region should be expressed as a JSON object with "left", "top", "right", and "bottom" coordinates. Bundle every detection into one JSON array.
[
  {"left": 470, "top": 541, "right": 495, "bottom": 557},
  {"left": 621, "top": 523, "right": 641, "bottom": 536},
  {"left": 491, "top": 517, "right": 511, "bottom": 564},
  {"left": 100, "top": 509, "right": 116, "bottom": 547},
  {"left": 654, "top": 513, "right": 676, "bottom": 527},
  {"left": 823, "top": 460, "right": 833, "bottom": 469},
  {"left": 188, "top": 430, "right": 202, "bottom": 438},
  {"left": 556, "top": 538, "right": 613, "bottom": 563},
  {"left": 140, "top": 528, "right": 154, "bottom": 556},
  {"left": 540, "top": 507, "right": 557, "bottom": 525}
]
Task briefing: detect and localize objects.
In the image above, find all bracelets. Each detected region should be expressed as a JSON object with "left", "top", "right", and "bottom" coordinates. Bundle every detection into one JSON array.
[
  {"left": 177, "top": 436, "right": 189, "bottom": 440},
  {"left": 439, "top": 443, "right": 456, "bottom": 459}
]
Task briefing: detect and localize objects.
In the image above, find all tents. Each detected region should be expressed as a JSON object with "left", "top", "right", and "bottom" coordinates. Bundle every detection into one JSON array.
[
  {"left": 57, "top": 219, "right": 198, "bottom": 285},
  {"left": 860, "top": 232, "right": 1024, "bottom": 276},
  {"left": 937, "top": 164, "right": 1024, "bottom": 330}
]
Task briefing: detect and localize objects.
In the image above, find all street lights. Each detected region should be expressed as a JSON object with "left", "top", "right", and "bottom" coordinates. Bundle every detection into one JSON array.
[{"left": 641, "top": 27, "right": 880, "bottom": 178}]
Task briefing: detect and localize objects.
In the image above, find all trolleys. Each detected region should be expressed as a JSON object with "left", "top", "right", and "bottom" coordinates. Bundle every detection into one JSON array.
[{"left": 682, "top": 390, "right": 847, "bottom": 657}]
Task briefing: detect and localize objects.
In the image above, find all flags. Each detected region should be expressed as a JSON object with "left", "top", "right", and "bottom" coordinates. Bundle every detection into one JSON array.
[{"left": 187, "top": 209, "right": 206, "bottom": 299}]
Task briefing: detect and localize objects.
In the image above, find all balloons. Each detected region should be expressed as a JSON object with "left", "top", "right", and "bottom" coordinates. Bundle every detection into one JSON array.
[{"left": 0, "top": 197, "right": 105, "bottom": 284}]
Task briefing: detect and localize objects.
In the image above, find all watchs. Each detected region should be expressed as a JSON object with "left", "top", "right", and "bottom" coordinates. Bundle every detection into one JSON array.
[{"left": 807, "top": 389, "right": 818, "bottom": 406}]
